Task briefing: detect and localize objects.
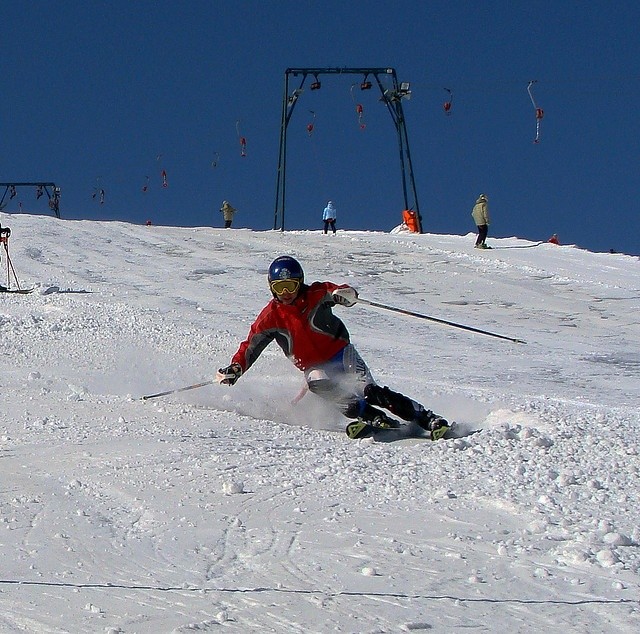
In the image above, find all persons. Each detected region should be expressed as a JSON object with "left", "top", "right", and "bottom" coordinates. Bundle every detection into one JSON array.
[
  {"left": 322, "top": 200, "right": 337, "bottom": 233},
  {"left": 218, "top": 254, "right": 448, "bottom": 431},
  {"left": 470, "top": 192, "right": 491, "bottom": 249},
  {"left": 219, "top": 200, "right": 238, "bottom": 228}
]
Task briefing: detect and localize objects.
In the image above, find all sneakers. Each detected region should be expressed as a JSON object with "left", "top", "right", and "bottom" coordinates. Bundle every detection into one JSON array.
[
  {"left": 373, "top": 414, "right": 400, "bottom": 428},
  {"left": 428, "top": 415, "right": 449, "bottom": 430}
]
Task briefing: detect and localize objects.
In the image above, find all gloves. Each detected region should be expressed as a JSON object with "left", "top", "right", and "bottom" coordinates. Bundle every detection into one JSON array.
[
  {"left": 217, "top": 364, "right": 243, "bottom": 386},
  {"left": 333, "top": 289, "right": 357, "bottom": 307}
]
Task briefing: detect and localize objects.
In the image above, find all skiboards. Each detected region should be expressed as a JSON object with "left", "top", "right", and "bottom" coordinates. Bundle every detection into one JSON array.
[
  {"left": 1, "top": 287, "right": 35, "bottom": 294},
  {"left": 347, "top": 420, "right": 457, "bottom": 442}
]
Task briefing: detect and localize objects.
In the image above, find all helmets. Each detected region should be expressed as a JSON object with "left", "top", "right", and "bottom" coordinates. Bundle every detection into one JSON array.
[{"left": 268, "top": 256, "right": 304, "bottom": 282}]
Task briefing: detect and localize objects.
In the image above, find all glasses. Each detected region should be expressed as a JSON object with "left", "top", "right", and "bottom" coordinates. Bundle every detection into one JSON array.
[{"left": 269, "top": 279, "right": 301, "bottom": 296}]
